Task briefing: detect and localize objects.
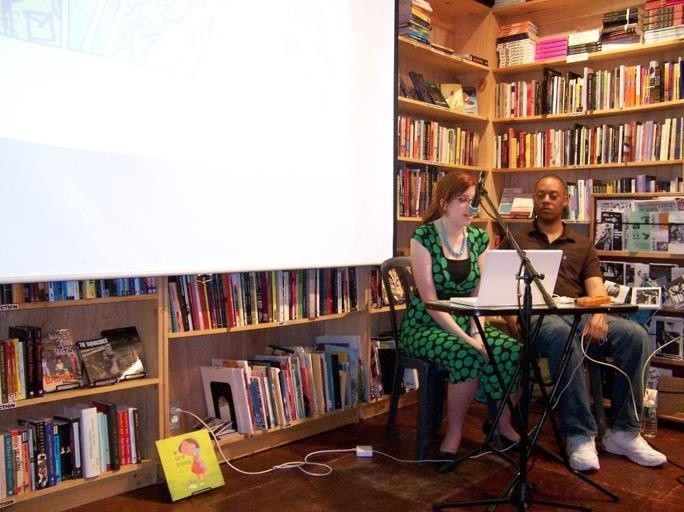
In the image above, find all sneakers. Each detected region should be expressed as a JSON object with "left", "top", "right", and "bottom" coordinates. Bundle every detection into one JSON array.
[
  {"left": 600, "top": 427, "right": 667, "bottom": 467},
  {"left": 565, "top": 434, "right": 600, "bottom": 471}
]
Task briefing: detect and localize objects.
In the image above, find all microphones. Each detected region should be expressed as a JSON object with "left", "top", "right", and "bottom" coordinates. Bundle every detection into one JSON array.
[{"left": 467, "top": 170, "right": 490, "bottom": 215}]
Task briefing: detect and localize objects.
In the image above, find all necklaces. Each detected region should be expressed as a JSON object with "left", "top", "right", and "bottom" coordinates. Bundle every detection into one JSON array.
[{"left": 439, "top": 220, "right": 468, "bottom": 257}]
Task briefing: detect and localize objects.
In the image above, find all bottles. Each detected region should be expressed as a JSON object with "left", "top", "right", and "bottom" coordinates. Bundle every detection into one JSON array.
[{"left": 644, "top": 389, "right": 658, "bottom": 437}]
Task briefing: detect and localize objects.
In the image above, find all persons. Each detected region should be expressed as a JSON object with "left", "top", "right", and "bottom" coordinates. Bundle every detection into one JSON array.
[
  {"left": 495, "top": 173, "right": 669, "bottom": 472},
  {"left": 394, "top": 171, "right": 535, "bottom": 473}
]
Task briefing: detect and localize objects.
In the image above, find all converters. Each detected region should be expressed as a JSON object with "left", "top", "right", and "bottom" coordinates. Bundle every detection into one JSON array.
[{"left": 355, "top": 445, "right": 373, "bottom": 457}]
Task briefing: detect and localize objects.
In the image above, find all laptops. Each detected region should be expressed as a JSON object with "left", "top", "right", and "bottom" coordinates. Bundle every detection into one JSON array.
[{"left": 449, "top": 249, "right": 563, "bottom": 307}]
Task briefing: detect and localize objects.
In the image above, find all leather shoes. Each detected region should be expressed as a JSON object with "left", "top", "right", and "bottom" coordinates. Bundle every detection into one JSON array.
[
  {"left": 433, "top": 438, "right": 458, "bottom": 474},
  {"left": 499, "top": 431, "right": 521, "bottom": 453}
]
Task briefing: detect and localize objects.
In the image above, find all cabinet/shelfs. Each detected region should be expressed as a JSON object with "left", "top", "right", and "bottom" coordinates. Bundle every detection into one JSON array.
[
  {"left": 0, "top": 1, "right": 493, "bottom": 509},
  {"left": 492, "top": 0, "right": 684, "bottom": 366}
]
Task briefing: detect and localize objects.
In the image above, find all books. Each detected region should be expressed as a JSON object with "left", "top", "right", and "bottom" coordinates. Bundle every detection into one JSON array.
[
  {"left": 398, "top": 1, "right": 433, "bottom": 47},
  {"left": 168, "top": 266, "right": 358, "bottom": 334},
  {"left": 398, "top": 115, "right": 480, "bottom": 167},
  {"left": 398, "top": 165, "right": 480, "bottom": 218},
  {"left": 1, "top": 276, "right": 159, "bottom": 305},
  {"left": 370, "top": 330, "right": 419, "bottom": 400},
  {"left": 497, "top": 187, "right": 534, "bottom": 219},
  {"left": 562, "top": 173, "right": 684, "bottom": 219},
  {"left": 496, "top": 1, "right": 683, "bottom": 68},
  {"left": 591, "top": 219, "right": 682, "bottom": 388},
  {"left": 495, "top": 56, "right": 682, "bottom": 119},
  {"left": 192, "top": 335, "right": 369, "bottom": 440},
  {"left": 155, "top": 428, "right": 225, "bottom": 502},
  {"left": 371, "top": 265, "right": 413, "bottom": 309},
  {"left": 494, "top": 116, "right": 682, "bottom": 170},
  {"left": 398, "top": 68, "right": 478, "bottom": 116},
  {"left": 2, "top": 326, "right": 146, "bottom": 404},
  {"left": 2, "top": 400, "right": 143, "bottom": 502},
  {"left": 431, "top": 43, "right": 488, "bottom": 67}
]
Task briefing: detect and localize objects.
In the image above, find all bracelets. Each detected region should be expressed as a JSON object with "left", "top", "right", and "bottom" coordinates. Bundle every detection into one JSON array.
[{"left": 470, "top": 328, "right": 486, "bottom": 334}]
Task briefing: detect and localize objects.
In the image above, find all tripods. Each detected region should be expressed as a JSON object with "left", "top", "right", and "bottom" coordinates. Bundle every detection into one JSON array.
[{"left": 432, "top": 190, "right": 592, "bottom": 512}]
